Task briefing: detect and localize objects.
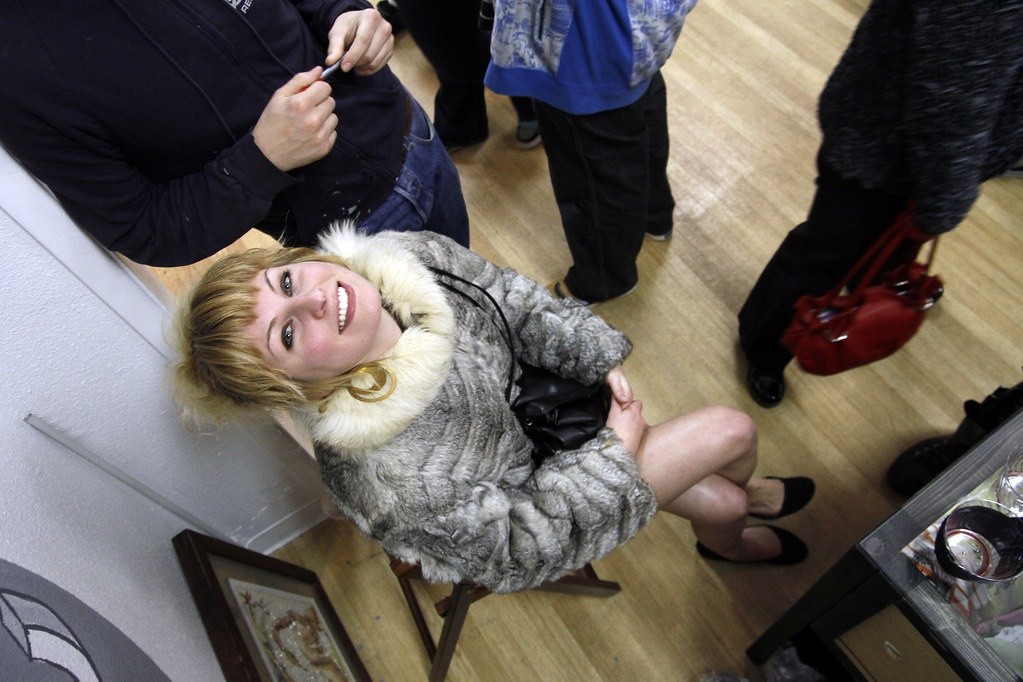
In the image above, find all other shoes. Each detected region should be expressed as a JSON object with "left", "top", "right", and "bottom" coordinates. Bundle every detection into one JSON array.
[
  {"left": 749, "top": 476, "right": 815, "bottom": 520},
  {"left": 431, "top": 123, "right": 485, "bottom": 152},
  {"left": 695, "top": 524, "right": 809, "bottom": 563},
  {"left": 643, "top": 228, "right": 672, "bottom": 242},
  {"left": 514, "top": 119, "right": 541, "bottom": 148},
  {"left": 543, "top": 277, "right": 638, "bottom": 309}
]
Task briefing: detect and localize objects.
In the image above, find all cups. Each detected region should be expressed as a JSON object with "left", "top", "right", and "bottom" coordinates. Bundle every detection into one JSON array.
[
  {"left": 934, "top": 498, "right": 1023, "bottom": 583},
  {"left": 994, "top": 443, "right": 1023, "bottom": 517}
]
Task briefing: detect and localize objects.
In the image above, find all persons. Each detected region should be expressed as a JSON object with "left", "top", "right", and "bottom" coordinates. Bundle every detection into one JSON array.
[
  {"left": 376, "top": 0, "right": 541, "bottom": 155},
  {"left": 0, "top": 0, "right": 469, "bottom": 268},
  {"left": 162, "top": 219, "right": 816, "bottom": 595},
  {"left": 481, "top": 0, "right": 698, "bottom": 311},
  {"left": 738, "top": 0, "right": 1023, "bottom": 409}
]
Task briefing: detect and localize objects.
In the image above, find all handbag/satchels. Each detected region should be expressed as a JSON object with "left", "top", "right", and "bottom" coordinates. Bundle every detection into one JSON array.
[
  {"left": 508, "top": 360, "right": 611, "bottom": 452},
  {"left": 788, "top": 215, "right": 946, "bottom": 376}
]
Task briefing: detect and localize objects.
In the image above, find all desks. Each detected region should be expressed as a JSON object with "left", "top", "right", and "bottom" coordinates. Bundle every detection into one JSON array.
[{"left": 747, "top": 415, "right": 1023, "bottom": 682}]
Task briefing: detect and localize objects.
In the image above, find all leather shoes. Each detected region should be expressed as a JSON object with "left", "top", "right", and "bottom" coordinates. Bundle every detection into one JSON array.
[{"left": 741, "top": 331, "right": 785, "bottom": 408}]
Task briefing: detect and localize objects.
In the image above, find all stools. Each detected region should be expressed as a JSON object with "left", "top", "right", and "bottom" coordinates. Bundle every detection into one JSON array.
[{"left": 383, "top": 546, "right": 622, "bottom": 682}]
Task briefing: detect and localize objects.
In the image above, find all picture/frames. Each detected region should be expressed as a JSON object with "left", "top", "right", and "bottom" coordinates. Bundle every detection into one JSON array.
[{"left": 169, "top": 527, "right": 372, "bottom": 682}]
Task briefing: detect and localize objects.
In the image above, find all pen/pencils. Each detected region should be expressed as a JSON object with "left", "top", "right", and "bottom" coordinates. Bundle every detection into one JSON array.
[{"left": 300, "top": 51, "right": 348, "bottom": 92}]
